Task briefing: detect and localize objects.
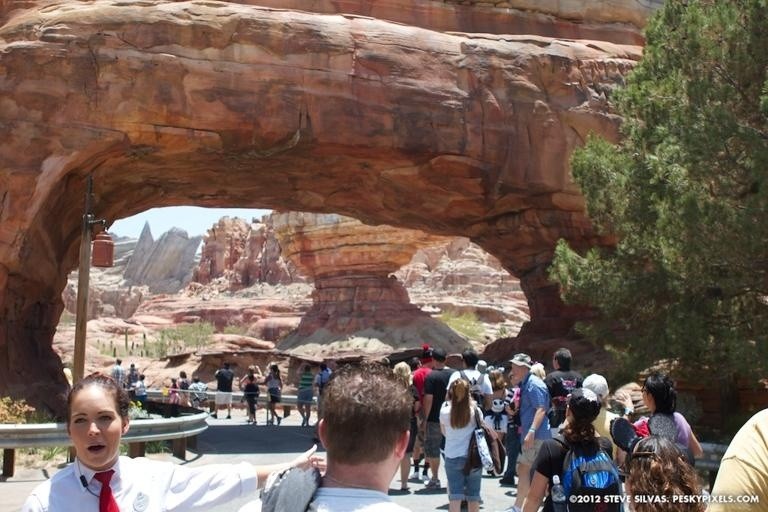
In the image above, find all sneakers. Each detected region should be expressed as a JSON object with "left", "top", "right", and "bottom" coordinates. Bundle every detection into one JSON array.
[
  {"left": 421, "top": 474, "right": 431, "bottom": 484},
  {"left": 268, "top": 419, "right": 274, "bottom": 424},
  {"left": 424, "top": 480, "right": 441, "bottom": 490},
  {"left": 211, "top": 414, "right": 218, "bottom": 419},
  {"left": 496, "top": 505, "right": 521, "bottom": 512},
  {"left": 410, "top": 471, "right": 420, "bottom": 480},
  {"left": 498, "top": 477, "right": 516, "bottom": 486},
  {"left": 277, "top": 417, "right": 282, "bottom": 425},
  {"left": 400, "top": 486, "right": 412, "bottom": 492},
  {"left": 302, "top": 416, "right": 308, "bottom": 426},
  {"left": 226, "top": 415, "right": 231, "bottom": 419}
]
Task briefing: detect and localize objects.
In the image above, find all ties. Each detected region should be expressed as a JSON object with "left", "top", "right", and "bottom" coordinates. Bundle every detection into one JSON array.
[{"left": 93, "top": 469, "right": 120, "bottom": 512}]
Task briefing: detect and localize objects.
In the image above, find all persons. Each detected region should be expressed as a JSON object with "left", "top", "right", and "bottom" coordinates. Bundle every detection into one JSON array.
[
  {"left": 23, "top": 370, "right": 326, "bottom": 512},
  {"left": 705, "top": 408, "right": 768, "bottom": 512},
  {"left": 167, "top": 348, "right": 710, "bottom": 512},
  {"left": 110, "top": 359, "right": 148, "bottom": 402}
]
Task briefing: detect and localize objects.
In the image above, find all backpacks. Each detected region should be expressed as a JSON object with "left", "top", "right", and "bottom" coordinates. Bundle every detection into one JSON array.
[
  {"left": 467, "top": 381, "right": 485, "bottom": 417},
  {"left": 180, "top": 382, "right": 189, "bottom": 390},
  {"left": 562, "top": 445, "right": 621, "bottom": 512}
]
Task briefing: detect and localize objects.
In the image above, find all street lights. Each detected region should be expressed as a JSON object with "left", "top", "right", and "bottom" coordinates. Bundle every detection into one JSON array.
[{"left": 64, "top": 174, "right": 114, "bottom": 385}]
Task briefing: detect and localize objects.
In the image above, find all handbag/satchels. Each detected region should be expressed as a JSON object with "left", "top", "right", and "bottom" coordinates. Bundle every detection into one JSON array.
[
  {"left": 474, "top": 426, "right": 494, "bottom": 474},
  {"left": 464, "top": 430, "right": 483, "bottom": 474}
]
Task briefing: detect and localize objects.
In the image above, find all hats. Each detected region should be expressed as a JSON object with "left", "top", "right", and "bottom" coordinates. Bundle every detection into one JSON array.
[
  {"left": 581, "top": 374, "right": 609, "bottom": 400},
  {"left": 418, "top": 350, "right": 433, "bottom": 359},
  {"left": 508, "top": 352, "right": 532, "bottom": 369},
  {"left": 567, "top": 388, "right": 603, "bottom": 412},
  {"left": 430, "top": 348, "right": 447, "bottom": 361}
]
